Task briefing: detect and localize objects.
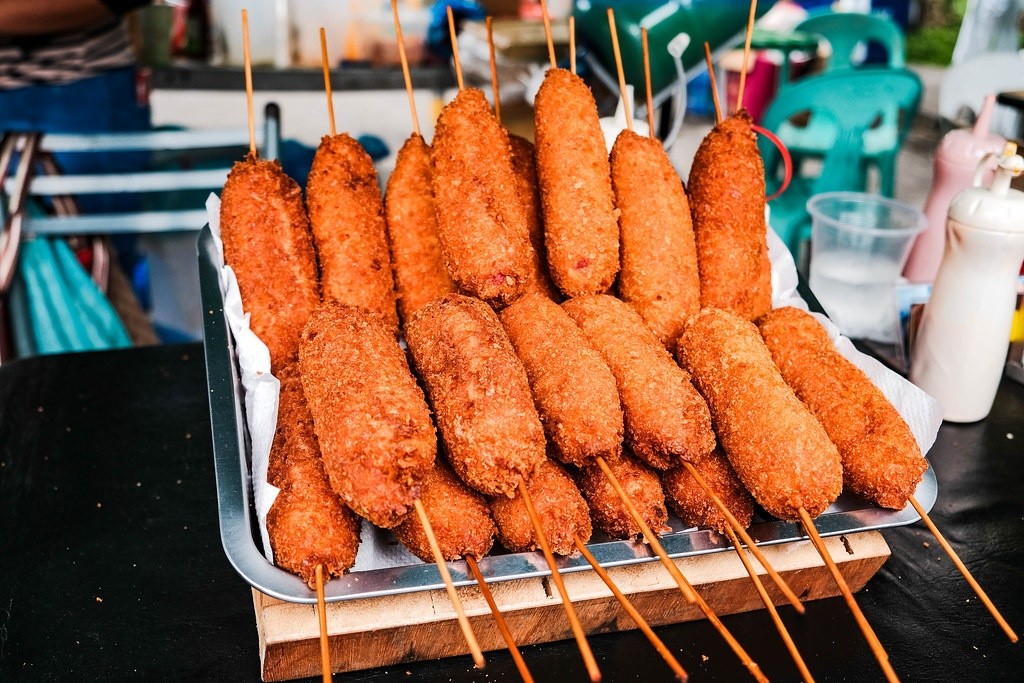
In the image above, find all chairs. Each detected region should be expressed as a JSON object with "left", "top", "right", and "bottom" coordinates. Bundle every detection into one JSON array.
[
  {"left": 772, "top": 12, "right": 909, "bottom": 201},
  {"left": 760, "top": 64, "right": 927, "bottom": 262}
]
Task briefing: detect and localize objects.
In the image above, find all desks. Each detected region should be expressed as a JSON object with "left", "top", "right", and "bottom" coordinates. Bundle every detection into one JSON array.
[
  {"left": 152, "top": 88, "right": 519, "bottom": 335},
  {"left": 0, "top": 335, "right": 1024, "bottom": 683}
]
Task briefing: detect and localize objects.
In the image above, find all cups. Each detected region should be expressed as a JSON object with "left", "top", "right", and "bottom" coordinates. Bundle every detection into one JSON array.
[{"left": 805, "top": 191, "right": 930, "bottom": 339}]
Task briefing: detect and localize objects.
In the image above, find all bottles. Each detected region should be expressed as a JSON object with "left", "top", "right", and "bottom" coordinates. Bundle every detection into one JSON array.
[
  {"left": 902, "top": 93, "right": 1009, "bottom": 283},
  {"left": 909, "top": 141, "right": 1024, "bottom": 424}
]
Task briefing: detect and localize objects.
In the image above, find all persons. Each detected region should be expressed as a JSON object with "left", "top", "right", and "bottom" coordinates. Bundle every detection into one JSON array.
[{"left": 0, "top": 0, "right": 200, "bottom": 349}]
